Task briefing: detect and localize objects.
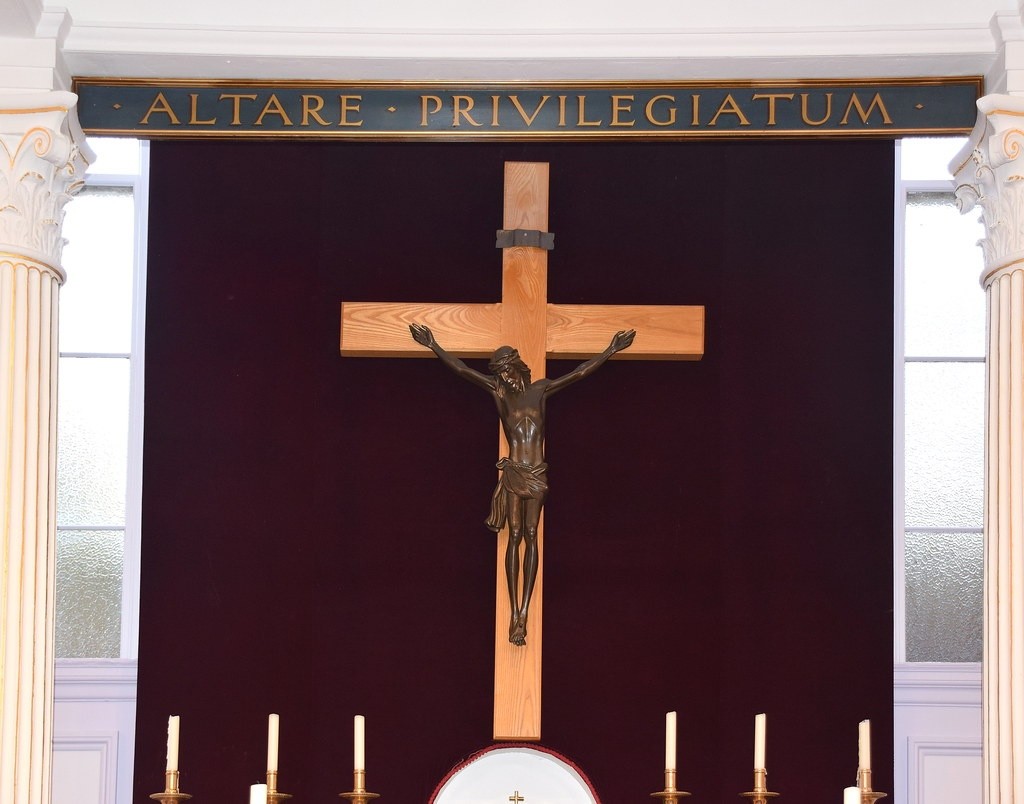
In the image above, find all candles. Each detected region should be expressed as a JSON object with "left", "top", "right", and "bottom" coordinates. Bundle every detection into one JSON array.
[
  {"left": 859, "top": 719, "right": 871, "bottom": 769},
  {"left": 266, "top": 713, "right": 279, "bottom": 772},
  {"left": 753, "top": 712, "right": 767, "bottom": 769},
  {"left": 355, "top": 714, "right": 364, "bottom": 771},
  {"left": 166, "top": 714, "right": 180, "bottom": 770},
  {"left": 665, "top": 710, "right": 676, "bottom": 769}
]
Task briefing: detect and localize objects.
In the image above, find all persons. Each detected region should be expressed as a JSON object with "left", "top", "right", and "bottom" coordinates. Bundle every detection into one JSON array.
[{"left": 408, "top": 323, "right": 636, "bottom": 646}]
